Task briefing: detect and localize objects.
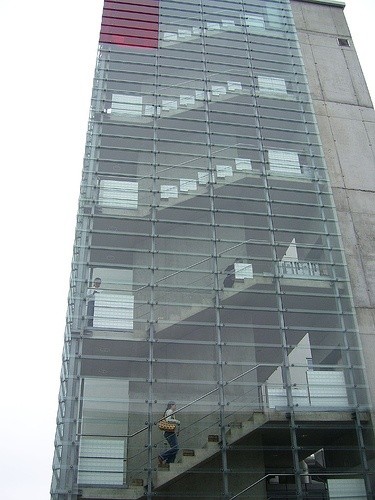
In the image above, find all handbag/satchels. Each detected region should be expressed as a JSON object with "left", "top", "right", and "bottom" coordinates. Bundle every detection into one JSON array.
[{"left": 159, "top": 420, "right": 177, "bottom": 432}]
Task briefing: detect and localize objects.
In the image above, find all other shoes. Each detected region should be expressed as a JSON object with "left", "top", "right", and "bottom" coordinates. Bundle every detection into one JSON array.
[{"left": 158, "top": 455, "right": 163, "bottom": 464}]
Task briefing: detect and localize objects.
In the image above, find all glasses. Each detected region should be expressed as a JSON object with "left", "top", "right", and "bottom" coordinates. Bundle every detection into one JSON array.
[{"left": 172, "top": 404, "right": 176, "bottom": 406}]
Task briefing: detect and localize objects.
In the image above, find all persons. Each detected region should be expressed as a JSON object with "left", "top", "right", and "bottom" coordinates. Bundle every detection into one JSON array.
[
  {"left": 85, "top": 278, "right": 102, "bottom": 324},
  {"left": 156, "top": 401, "right": 180, "bottom": 465},
  {"left": 224, "top": 257, "right": 242, "bottom": 289}
]
